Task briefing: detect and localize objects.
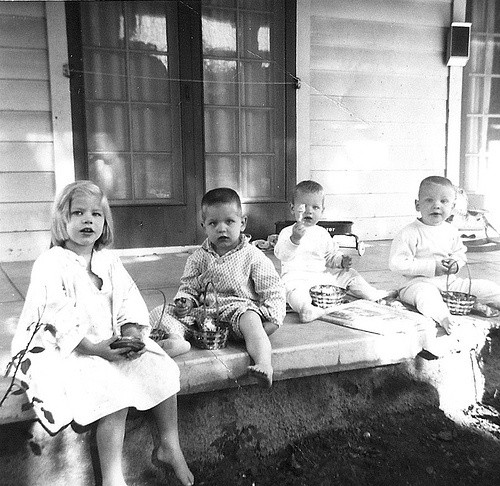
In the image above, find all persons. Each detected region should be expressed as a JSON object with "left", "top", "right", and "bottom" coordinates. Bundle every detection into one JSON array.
[
  {"left": 148, "top": 187, "right": 286, "bottom": 389},
  {"left": 273, "top": 180, "right": 399, "bottom": 323},
  {"left": 389, "top": 175, "right": 500, "bottom": 335},
  {"left": 9, "top": 179, "right": 194, "bottom": 486}
]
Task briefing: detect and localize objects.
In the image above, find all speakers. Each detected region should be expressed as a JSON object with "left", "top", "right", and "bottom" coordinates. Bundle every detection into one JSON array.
[{"left": 446, "top": 22, "right": 471, "bottom": 67}]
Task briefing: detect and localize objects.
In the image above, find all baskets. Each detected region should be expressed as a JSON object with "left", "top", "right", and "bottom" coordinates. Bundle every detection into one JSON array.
[
  {"left": 189, "top": 281, "right": 231, "bottom": 350},
  {"left": 440, "top": 259, "right": 477, "bottom": 316},
  {"left": 139, "top": 288, "right": 166, "bottom": 341},
  {"left": 309, "top": 284, "right": 346, "bottom": 309}
]
